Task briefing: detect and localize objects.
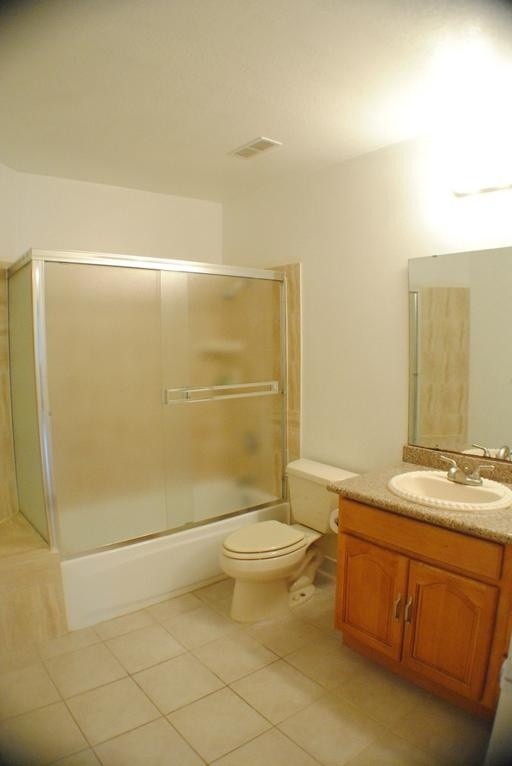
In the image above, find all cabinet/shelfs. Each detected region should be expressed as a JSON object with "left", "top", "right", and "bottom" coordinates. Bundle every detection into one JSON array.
[{"left": 334, "top": 496, "right": 511, "bottom": 721}]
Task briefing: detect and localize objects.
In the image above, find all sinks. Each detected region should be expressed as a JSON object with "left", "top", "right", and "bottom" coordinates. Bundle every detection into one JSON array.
[
  {"left": 388, "top": 470, "right": 512, "bottom": 510},
  {"left": 465, "top": 447, "right": 510, "bottom": 460}
]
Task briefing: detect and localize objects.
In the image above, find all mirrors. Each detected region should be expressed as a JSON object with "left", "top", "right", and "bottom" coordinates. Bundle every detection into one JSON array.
[{"left": 402, "top": 246, "right": 512, "bottom": 484}]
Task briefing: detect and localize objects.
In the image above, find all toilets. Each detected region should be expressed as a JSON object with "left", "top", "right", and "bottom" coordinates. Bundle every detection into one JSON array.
[{"left": 218, "top": 458, "right": 360, "bottom": 623}]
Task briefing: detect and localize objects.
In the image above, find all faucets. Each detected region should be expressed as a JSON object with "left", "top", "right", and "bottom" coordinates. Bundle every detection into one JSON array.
[
  {"left": 447, "top": 465, "right": 467, "bottom": 482},
  {"left": 496, "top": 446, "right": 511, "bottom": 461}
]
